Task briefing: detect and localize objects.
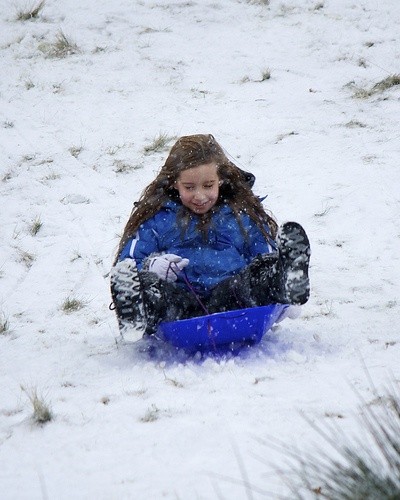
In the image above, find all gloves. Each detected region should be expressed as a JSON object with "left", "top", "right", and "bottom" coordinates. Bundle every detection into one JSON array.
[{"left": 149, "top": 252, "right": 190, "bottom": 284}]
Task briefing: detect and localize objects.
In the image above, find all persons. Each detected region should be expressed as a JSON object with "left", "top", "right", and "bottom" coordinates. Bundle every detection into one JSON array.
[{"left": 109, "top": 134, "right": 312, "bottom": 343}]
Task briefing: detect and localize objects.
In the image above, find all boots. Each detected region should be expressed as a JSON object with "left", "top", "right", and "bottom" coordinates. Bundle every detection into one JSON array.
[
  {"left": 239, "top": 222, "right": 312, "bottom": 312},
  {"left": 137, "top": 270, "right": 206, "bottom": 337}
]
